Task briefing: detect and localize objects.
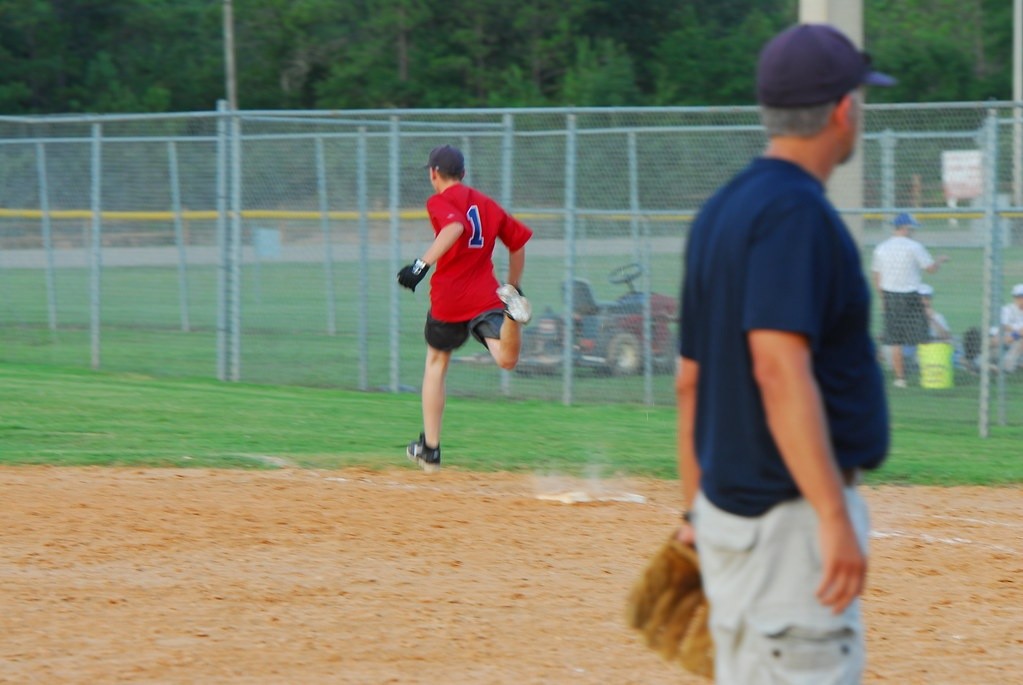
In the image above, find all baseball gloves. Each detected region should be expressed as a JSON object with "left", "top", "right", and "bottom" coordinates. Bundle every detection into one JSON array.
[{"left": 622, "top": 517, "right": 716, "bottom": 680}]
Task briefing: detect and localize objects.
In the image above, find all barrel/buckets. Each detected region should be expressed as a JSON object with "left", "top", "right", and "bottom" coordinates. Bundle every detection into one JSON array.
[{"left": 917, "top": 342, "right": 953, "bottom": 388}]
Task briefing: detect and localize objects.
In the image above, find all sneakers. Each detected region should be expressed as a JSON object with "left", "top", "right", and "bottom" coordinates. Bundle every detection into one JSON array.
[
  {"left": 495, "top": 283, "right": 531, "bottom": 324},
  {"left": 407, "top": 433, "right": 441, "bottom": 470}
]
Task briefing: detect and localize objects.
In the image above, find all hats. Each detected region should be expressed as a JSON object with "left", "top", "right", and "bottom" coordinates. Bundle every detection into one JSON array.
[
  {"left": 1010, "top": 284, "right": 1023, "bottom": 297},
  {"left": 917, "top": 284, "right": 933, "bottom": 295},
  {"left": 895, "top": 212, "right": 920, "bottom": 228},
  {"left": 757, "top": 25, "right": 899, "bottom": 108},
  {"left": 423, "top": 144, "right": 464, "bottom": 173}
]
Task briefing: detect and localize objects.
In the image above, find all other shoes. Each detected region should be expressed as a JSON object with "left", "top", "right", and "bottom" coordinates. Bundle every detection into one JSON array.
[{"left": 895, "top": 379, "right": 905, "bottom": 387}]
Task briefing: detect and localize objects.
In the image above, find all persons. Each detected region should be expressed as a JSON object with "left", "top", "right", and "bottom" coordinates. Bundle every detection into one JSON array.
[
  {"left": 675, "top": 23, "right": 898, "bottom": 684},
  {"left": 1000, "top": 283, "right": 1023, "bottom": 372},
  {"left": 875, "top": 284, "right": 961, "bottom": 365},
  {"left": 871, "top": 212, "right": 950, "bottom": 388},
  {"left": 974, "top": 327, "right": 1005, "bottom": 369},
  {"left": 396, "top": 144, "right": 535, "bottom": 473}
]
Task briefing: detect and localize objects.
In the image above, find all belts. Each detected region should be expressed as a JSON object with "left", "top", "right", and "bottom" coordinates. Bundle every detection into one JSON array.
[{"left": 842, "top": 466, "right": 858, "bottom": 487}]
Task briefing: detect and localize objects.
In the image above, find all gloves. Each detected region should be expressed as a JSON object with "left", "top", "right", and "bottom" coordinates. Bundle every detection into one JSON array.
[{"left": 396, "top": 258, "right": 430, "bottom": 292}]
